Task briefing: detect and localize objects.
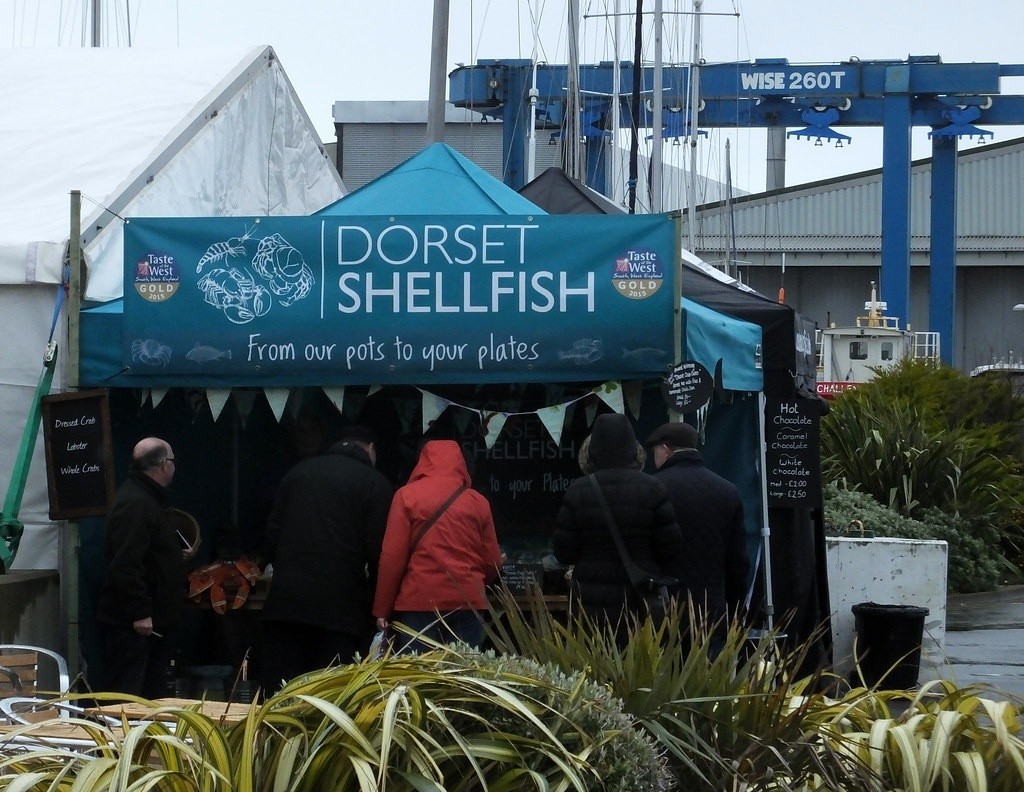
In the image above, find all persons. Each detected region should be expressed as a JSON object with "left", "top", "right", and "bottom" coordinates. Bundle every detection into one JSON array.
[
  {"left": 372, "top": 439, "right": 507, "bottom": 655},
  {"left": 272, "top": 404, "right": 330, "bottom": 473},
  {"left": 260, "top": 425, "right": 394, "bottom": 688},
  {"left": 97, "top": 436, "right": 195, "bottom": 696},
  {"left": 552, "top": 414, "right": 749, "bottom": 672}
]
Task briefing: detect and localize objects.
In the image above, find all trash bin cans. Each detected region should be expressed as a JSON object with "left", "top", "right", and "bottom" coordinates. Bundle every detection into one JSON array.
[{"left": 852, "top": 600, "right": 932, "bottom": 699}]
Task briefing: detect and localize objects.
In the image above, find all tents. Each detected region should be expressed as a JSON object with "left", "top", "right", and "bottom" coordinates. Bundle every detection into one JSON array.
[
  {"left": 0, "top": 43, "right": 350, "bottom": 570},
  {"left": 69, "top": 139, "right": 774, "bottom": 709},
  {"left": 517, "top": 166, "right": 817, "bottom": 401}
]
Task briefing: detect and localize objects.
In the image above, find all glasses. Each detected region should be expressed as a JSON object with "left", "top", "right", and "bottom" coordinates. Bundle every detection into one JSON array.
[{"left": 166, "top": 457, "right": 177, "bottom": 463}]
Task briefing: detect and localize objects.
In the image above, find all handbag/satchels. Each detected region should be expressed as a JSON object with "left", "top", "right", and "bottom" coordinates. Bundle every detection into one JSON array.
[
  {"left": 369, "top": 627, "right": 388, "bottom": 665},
  {"left": 647, "top": 566, "right": 691, "bottom": 635}
]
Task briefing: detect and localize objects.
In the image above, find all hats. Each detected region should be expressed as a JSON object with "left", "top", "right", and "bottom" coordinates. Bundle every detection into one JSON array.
[
  {"left": 645, "top": 421, "right": 699, "bottom": 448},
  {"left": 331, "top": 423, "right": 382, "bottom": 446}
]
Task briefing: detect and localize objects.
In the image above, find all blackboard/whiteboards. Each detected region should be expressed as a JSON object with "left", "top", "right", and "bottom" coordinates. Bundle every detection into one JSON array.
[
  {"left": 39, "top": 387, "right": 117, "bottom": 520},
  {"left": 768, "top": 398, "right": 819, "bottom": 509}
]
